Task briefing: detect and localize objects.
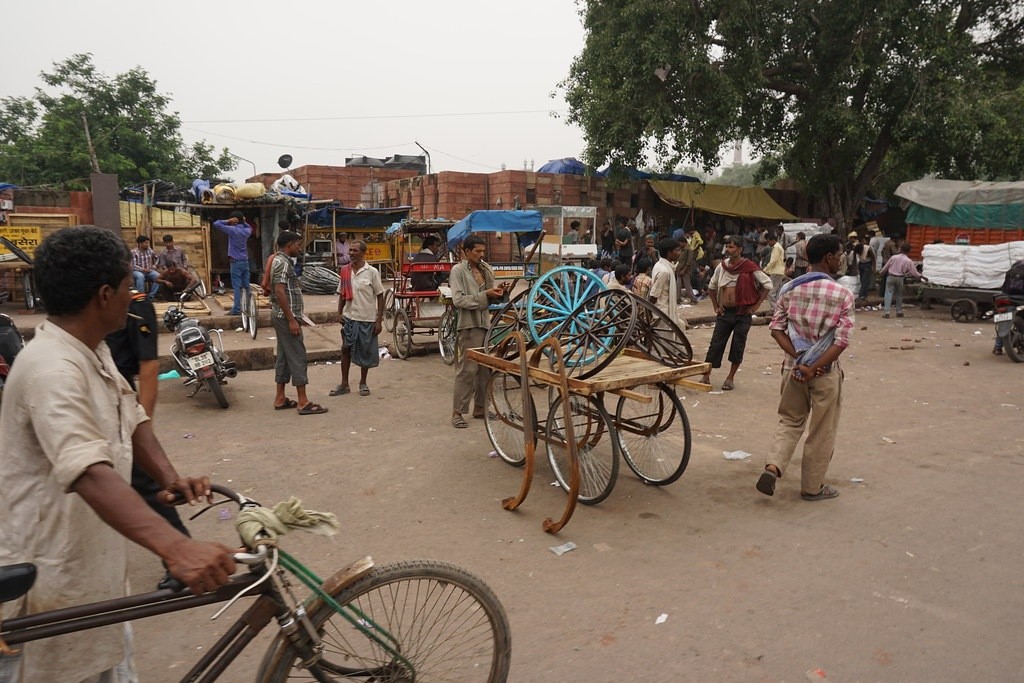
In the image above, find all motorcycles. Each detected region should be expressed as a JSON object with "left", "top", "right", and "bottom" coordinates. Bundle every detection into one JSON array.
[{"left": 159, "top": 277, "right": 239, "bottom": 410}]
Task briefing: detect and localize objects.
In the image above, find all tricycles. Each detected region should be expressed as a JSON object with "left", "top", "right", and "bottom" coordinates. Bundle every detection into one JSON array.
[{"left": 383, "top": 216, "right": 458, "bottom": 366}]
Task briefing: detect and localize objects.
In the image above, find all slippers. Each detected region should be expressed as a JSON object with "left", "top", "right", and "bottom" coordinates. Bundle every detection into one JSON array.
[
  {"left": 473, "top": 411, "right": 498, "bottom": 419},
  {"left": 451, "top": 414, "right": 468, "bottom": 429},
  {"left": 721, "top": 378, "right": 734, "bottom": 390},
  {"left": 358, "top": 383, "right": 370, "bottom": 396},
  {"left": 757, "top": 465, "right": 777, "bottom": 496},
  {"left": 329, "top": 385, "right": 350, "bottom": 396},
  {"left": 275, "top": 397, "right": 297, "bottom": 410},
  {"left": 801, "top": 485, "right": 838, "bottom": 500},
  {"left": 299, "top": 400, "right": 329, "bottom": 413}
]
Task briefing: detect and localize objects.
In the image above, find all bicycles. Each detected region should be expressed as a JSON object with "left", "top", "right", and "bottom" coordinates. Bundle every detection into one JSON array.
[{"left": 0, "top": 484, "right": 512, "bottom": 683}]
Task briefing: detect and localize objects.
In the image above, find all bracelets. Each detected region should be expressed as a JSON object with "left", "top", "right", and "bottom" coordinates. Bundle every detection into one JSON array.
[{"left": 286, "top": 313, "right": 294, "bottom": 321}]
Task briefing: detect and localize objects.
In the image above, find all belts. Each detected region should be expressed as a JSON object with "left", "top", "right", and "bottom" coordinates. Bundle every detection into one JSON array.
[{"left": 890, "top": 273, "right": 903, "bottom": 277}]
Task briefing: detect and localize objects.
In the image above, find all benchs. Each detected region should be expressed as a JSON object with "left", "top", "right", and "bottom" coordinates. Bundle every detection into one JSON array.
[
  {"left": 393, "top": 262, "right": 459, "bottom": 298},
  {"left": 489, "top": 276, "right": 538, "bottom": 310}
]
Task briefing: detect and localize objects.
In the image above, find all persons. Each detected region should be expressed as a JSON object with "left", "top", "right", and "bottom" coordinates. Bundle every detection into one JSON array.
[
  {"left": 794, "top": 231, "right": 809, "bottom": 276},
  {"left": 213, "top": 210, "right": 253, "bottom": 315},
  {"left": 564, "top": 213, "right": 728, "bottom": 359},
  {"left": 756, "top": 234, "right": 855, "bottom": 501},
  {"left": 269, "top": 228, "right": 328, "bottom": 415},
  {"left": 128, "top": 233, "right": 161, "bottom": 301},
  {"left": 448, "top": 235, "right": 511, "bottom": 428},
  {"left": 698, "top": 235, "right": 773, "bottom": 391},
  {"left": 739, "top": 219, "right": 793, "bottom": 316},
  {"left": 842, "top": 231, "right": 904, "bottom": 301},
  {"left": 103, "top": 242, "right": 160, "bottom": 424},
  {"left": 158, "top": 232, "right": 188, "bottom": 270},
  {"left": 336, "top": 231, "right": 353, "bottom": 267},
  {"left": 153, "top": 260, "right": 196, "bottom": 302},
  {"left": 410, "top": 235, "right": 450, "bottom": 291},
  {"left": 0, "top": 226, "right": 248, "bottom": 683},
  {"left": 993, "top": 260, "right": 1024, "bottom": 354},
  {"left": 328, "top": 240, "right": 386, "bottom": 396},
  {"left": 878, "top": 243, "right": 928, "bottom": 320},
  {"left": 820, "top": 215, "right": 835, "bottom": 234}
]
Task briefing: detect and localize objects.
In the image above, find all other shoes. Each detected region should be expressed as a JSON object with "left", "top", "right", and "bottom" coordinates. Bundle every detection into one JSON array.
[
  {"left": 882, "top": 313, "right": 891, "bottom": 319},
  {"left": 224, "top": 310, "right": 240, "bottom": 316},
  {"left": 896, "top": 313, "right": 903, "bottom": 318},
  {"left": 991, "top": 347, "right": 1003, "bottom": 354},
  {"left": 157, "top": 573, "right": 179, "bottom": 593}
]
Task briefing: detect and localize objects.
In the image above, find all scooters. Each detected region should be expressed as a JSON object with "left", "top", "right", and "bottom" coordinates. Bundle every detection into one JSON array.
[{"left": 993, "top": 291, "right": 1024, "bottom": 364}]
